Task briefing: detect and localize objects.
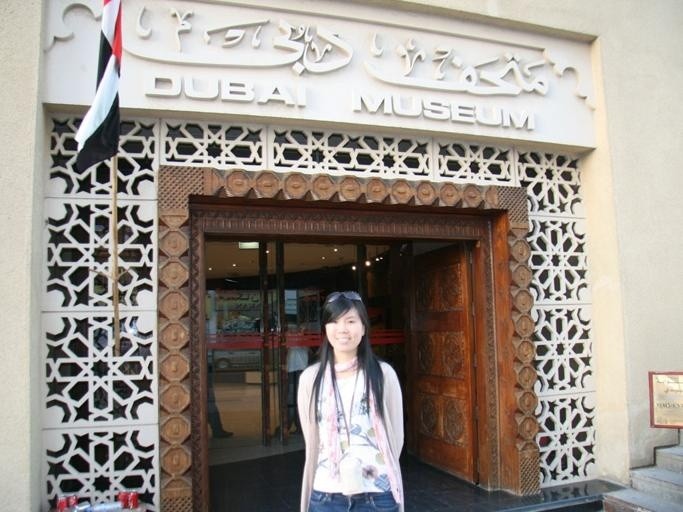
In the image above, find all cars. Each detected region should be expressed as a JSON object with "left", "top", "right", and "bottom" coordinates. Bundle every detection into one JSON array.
[{"left": 205, "top": 350, "right": 261, "bottom": 373}]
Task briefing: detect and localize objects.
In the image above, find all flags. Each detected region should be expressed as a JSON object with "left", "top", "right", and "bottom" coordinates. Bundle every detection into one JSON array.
[{"left": 68, "top": 0, "right": 122, "bottom": 182}]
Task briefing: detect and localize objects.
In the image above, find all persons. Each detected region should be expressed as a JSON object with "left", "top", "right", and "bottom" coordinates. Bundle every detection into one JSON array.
[
  {"left": 296, "top": 291, "right": 405, "bottom": 512},
  {"left": 208, "top": 362, "right": 233, "bottom": 437},
  {"left": 284, "top": 345, "right": 309, "bottom": 435}
]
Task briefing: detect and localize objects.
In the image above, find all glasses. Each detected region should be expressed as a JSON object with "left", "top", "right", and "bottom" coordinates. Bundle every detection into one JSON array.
[{"left": 325, "top": 290, "right": 363, "bottom": 304}]
